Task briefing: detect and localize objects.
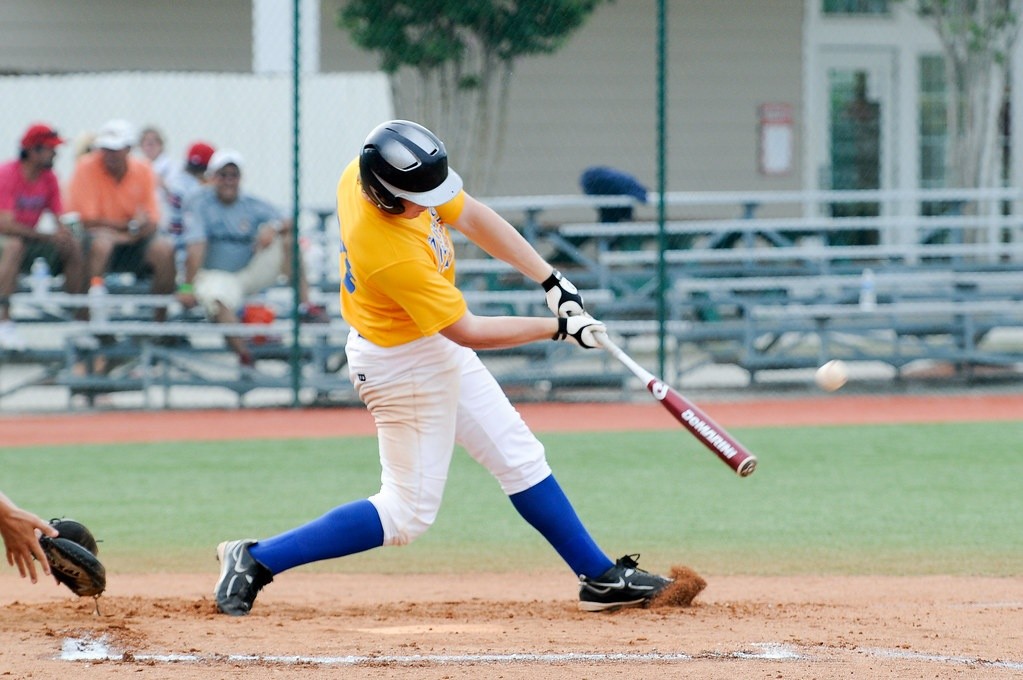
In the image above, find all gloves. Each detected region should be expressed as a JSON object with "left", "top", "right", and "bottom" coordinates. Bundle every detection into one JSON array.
[
  {"left": 543, "top": 269, "right": 584, "bottom": 318},
  {"left": 552, "top": 316, "right": 608, "bottom": 350}
]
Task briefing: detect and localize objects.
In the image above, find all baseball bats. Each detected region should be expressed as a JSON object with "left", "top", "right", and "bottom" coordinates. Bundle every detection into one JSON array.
[{"left": 571, "top": 310, "right": 758, "bottom": 479}]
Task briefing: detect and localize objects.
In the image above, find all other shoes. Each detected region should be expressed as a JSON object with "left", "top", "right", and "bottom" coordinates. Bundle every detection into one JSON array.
[
  {"left": 306, "top": 305, "right": 330, "bottom": 324},
  {"left": 241, "top": 352, "right": 255, "bottom": 369}
]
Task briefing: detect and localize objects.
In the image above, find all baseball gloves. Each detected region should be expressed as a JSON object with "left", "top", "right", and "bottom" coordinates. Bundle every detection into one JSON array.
[{"left": 38, "top": 518, "right": 107, "bottom": 598}]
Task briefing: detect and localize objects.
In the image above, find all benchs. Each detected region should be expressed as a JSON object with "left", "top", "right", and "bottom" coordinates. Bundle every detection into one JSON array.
[{"left": 3, "top": 177, "right": 1023, "bottom": 410}]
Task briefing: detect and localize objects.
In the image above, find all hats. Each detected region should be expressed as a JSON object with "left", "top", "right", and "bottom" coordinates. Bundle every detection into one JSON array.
[
  {"left": 94, "top": 120, "right": 135, "bottom": 150},
  {"left": 22, "top": 125, "right": 63, "bottom": 150},
  {"left": 208, "top": 151, "right": 240, "bottom": 177},
  {"left": 189, "top": 144, "right": 214, "bottom": 166}
]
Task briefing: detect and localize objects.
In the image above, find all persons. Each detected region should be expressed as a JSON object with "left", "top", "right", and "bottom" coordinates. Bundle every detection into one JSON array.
[
  {"left": 0, "top": 493, "right": 59, "bottom": 584},
  {"left": 213, "top": 119, "right": 672, "bottom": 618},
  {"left": 0, "top": 119, "right": 327, "bottom": 378}
]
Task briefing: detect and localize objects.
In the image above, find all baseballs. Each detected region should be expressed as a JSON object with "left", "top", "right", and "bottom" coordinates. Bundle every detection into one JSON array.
[{"left": 814, "top": 359, "right": 849, "bottom": 394}]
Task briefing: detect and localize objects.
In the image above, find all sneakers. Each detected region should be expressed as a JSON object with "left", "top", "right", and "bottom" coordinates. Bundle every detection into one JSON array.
[
  {"left": 578, "top": 553, "right": 675, "bottom": 612},
  {"left": 213, "top": 539, "right": 274, "bottom": 616}
]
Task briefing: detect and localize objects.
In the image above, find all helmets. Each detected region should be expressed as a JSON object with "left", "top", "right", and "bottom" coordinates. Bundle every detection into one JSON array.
[{"left": 360, "top": 120, "right": 463, "bottom": 214}]
